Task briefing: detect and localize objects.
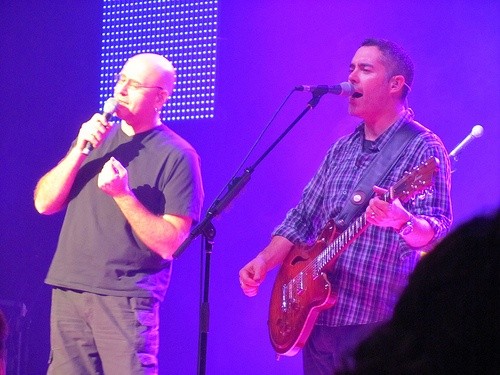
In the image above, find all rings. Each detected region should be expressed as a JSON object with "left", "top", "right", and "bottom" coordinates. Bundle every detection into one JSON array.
[{"left": 240, "top": 282, "right": 244, "bottom": 287}]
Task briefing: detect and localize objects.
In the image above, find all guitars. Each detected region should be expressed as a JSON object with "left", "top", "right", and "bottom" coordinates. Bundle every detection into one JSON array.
[{"left": 266, "top": 156, "right": 441, "bottom": 358}]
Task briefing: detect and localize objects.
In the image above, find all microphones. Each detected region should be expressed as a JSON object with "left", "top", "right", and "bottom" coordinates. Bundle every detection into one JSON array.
[
  {"left": 81, "top": 97, "right": 119, "bottom": 156},
  {"left": 295, "top": 82, "right": 355, "bottom": 97}
]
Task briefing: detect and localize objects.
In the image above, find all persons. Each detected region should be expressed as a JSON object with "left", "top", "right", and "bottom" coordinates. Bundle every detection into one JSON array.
[
  {"left": 34, "top": 53, "right": 204, "bottom": 375},
  {"left": 239, "top": 37, "right": 453, "bottom": 375}
]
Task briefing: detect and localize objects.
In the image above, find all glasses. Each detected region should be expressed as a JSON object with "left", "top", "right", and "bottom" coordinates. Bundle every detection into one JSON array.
[{"left": 114, "top": 73, "right": 163, "bottom": 91}]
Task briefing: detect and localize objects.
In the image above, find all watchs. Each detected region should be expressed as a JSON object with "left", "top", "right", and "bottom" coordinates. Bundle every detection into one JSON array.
[{"left": 394, "top": 214, "right": 415, "bottom": 236}]
